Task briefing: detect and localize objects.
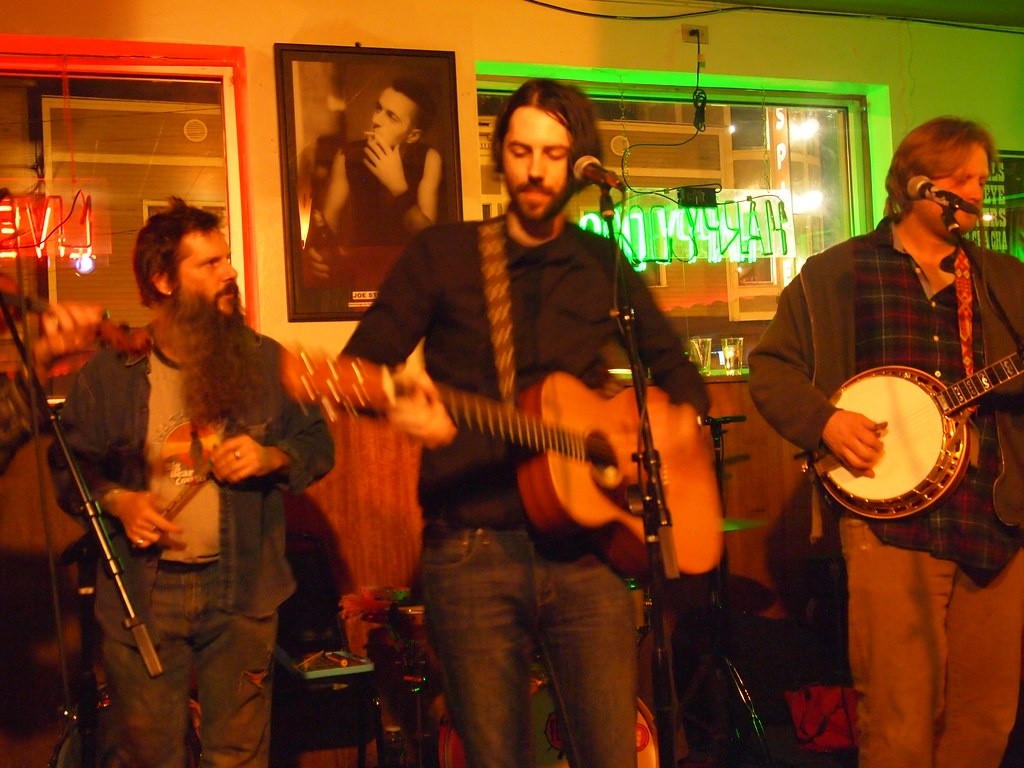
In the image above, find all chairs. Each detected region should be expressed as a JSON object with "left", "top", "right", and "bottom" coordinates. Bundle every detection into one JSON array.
[
  {"left": 709, "top": 604, "right": 859, "bottom": 768},
  {"left": 270, "top": 531, "right": 383, "bottom": 768}
]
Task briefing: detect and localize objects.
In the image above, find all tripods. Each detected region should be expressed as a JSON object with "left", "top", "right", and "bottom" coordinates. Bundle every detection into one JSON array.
[{"left": 680, "top": 416, "right": 776, "bottom": 768}]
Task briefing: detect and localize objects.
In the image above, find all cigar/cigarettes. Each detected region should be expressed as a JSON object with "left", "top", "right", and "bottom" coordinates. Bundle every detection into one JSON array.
[{"left": 361, "top": 130, "right": 379, "bottom": 137}]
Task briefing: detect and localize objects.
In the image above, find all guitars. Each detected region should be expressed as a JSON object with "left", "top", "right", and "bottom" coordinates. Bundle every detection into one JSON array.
[{"left": 282, "top": 341, "right": 725, "bottom": 583}]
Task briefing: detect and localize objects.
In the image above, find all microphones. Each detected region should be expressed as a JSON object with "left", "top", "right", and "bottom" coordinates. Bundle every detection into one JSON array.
[
  {"left": 908, "top": 174, "right": 980, "bottom": 215},
  {"left": 573, "top": 154, "right": 627, "bottom": 193}
]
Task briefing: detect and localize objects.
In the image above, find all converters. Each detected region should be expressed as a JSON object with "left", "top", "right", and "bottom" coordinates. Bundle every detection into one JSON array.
[{"left": 678, "top": 186, "right": 717, "bottom": 209}]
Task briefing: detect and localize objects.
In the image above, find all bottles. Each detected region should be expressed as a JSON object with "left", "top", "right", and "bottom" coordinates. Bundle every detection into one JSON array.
[
  {"left": 385, "top": 726, "right": 408, "bottom": 768},
  {"left": 312, "top": 210, "right": 342, "bottom": 285}
]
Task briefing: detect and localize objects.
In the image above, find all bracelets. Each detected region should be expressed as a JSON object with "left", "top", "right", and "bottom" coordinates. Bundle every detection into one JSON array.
[
  {"left": 102, "top": 482, "right": 122, "bottom": 518},
  {"left": 390, "top": 189, "right": 417, "bottom": 213},
  {"left": 687, "top": 405, "right": 707, "bottom": 442}
]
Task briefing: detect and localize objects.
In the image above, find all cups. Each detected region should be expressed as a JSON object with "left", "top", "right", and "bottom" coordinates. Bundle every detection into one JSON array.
[
  {"left": 690, "top": 338, "right": 711, "bottom": 374},
  {"left": 721, "top": 337, "right": 743, "bottom": 373}
]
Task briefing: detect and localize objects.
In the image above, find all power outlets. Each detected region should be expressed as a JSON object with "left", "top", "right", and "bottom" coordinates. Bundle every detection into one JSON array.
[{"left": 681, "top": 22, "right": 709, "bottom": 44}]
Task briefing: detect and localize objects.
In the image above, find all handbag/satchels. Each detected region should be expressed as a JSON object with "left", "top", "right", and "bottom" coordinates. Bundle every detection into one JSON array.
[{"left": 783, "top": 679, "right": 858, "bottom": 751}]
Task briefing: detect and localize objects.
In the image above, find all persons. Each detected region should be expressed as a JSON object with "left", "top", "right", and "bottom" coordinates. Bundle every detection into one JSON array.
[
  {"left": 297, "top": 78, "right": 445, "bottom": 290},
  {"left": 47, "top": 203, "right": 336, "bottom": 768},
  {"left": 742, "top": 115, "right": 1023, "bottom": 768},
  {"left": 335, "top": 81, "right": 714, "bottom": 768}
]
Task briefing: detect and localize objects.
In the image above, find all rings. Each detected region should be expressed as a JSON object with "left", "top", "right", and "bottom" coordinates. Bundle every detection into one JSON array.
[
  {"left": 136, "top": 539, "right": 145, "bottom": 546},
  {"left": 233, "top": 447, "right": 241, "bottom": 458}
]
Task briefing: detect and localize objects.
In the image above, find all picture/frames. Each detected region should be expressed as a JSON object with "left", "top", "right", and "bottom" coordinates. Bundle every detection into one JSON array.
[{"left": 274, "top": 42, "right": 463, "bottom": 322}]
